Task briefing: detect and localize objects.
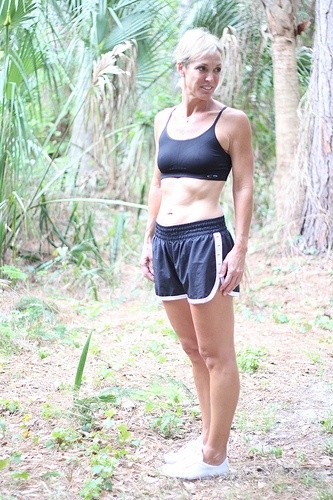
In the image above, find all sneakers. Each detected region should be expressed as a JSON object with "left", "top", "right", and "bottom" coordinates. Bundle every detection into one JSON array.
[{"left": 159, "top": 436, "right": 231, "bottom": 481}]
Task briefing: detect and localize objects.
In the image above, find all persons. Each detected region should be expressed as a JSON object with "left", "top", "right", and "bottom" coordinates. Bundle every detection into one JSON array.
[{"left": 143, "top": 26, "right": 254, "bottom": 475}]
